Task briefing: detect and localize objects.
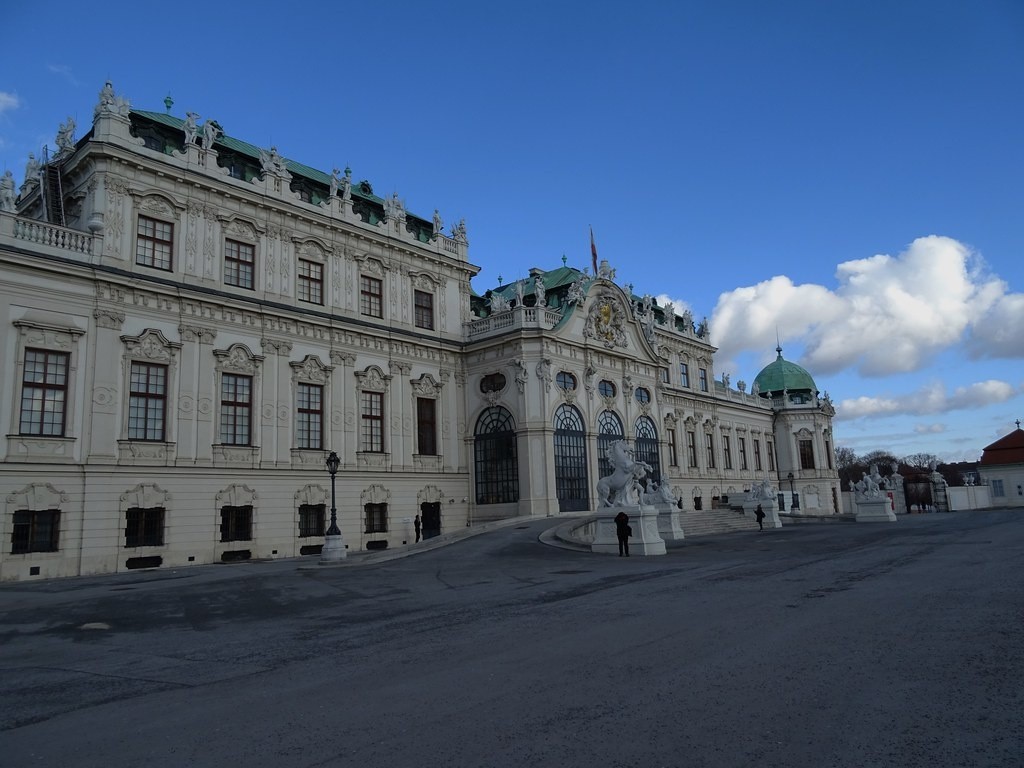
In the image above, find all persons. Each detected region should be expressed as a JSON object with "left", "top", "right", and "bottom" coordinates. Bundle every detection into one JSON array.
[
  {"left": 183, "top": 113, "right": 218, "bottom": 149},
  {"left": 512, "top": 279, "right": 545, "bottom": 307},
  {"left": 753, "top": 482, "right": 760, "bottom": 499},
  {"left": 624, "top": 285, "right": 708, "bottom": 336},
  {"left": 646, "top": 479, "right": 655, "bottom": 494},
  {"left": 414, "top": 515, "right": 421, "bottom": 542},
  {"left": 753, "top": 504, "right": 766, "bottom": 531},
  {"left": 753, "top": 382, "right": 760, "bottom": 395},
  {"left": 614, "top": 512, "right": 630, "bottom": 558},
  {"left": 0, "top": 116, "right": 74, "bottom": 212},
  {"left": 784, "top": 387, "right": 788, "bottom": 396},
  {"left": 331, "top": 169, "right": 352, "bottom": 199},
  {"left": 722, "top": 372, "right": 730, "bottom": 385},
  {"left": 433, "top": 209, "right": 443, "bottom": 234},
  {"left": 862, "top": 472, "right": 879, "bottom": 499},
  {"left": 633, "top": 460, "right": 646, "bottom": 505}
]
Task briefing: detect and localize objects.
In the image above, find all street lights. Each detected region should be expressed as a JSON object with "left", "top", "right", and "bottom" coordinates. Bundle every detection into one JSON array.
[
  {"left": 787, "top": 472, "right": 802, "bottom": 515},
  {"left": 321, "top": 451, "right": 347, "bottom": 561}
]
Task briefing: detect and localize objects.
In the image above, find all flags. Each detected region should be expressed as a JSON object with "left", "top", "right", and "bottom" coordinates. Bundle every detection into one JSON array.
[{"left": 591, "top": 229, "right": 597, "bottom": 274}]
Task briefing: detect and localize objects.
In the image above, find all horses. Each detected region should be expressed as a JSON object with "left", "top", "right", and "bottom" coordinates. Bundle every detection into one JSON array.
[
  {"left": 596, "top": 439, "right": 653, "bottom": 510},
  {"left": 853, "top": 464, "right": 891, "bottom": 499}
]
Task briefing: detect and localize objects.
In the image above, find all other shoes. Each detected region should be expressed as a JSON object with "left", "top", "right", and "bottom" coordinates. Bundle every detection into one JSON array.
[
  {"left": 626, "top": 554, "right": 629, "bottom": 557},
  {"left": 619, "top": 554, "right": 622, "bottom": 557}
]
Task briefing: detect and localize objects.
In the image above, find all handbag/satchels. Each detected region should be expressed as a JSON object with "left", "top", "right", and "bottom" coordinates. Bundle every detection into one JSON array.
[
  {"left": 627, "top": 526, "right": 632, "bottom": 536},
  {"left": 761, "top": 512, "right": 765, "bottom": 518}
]
